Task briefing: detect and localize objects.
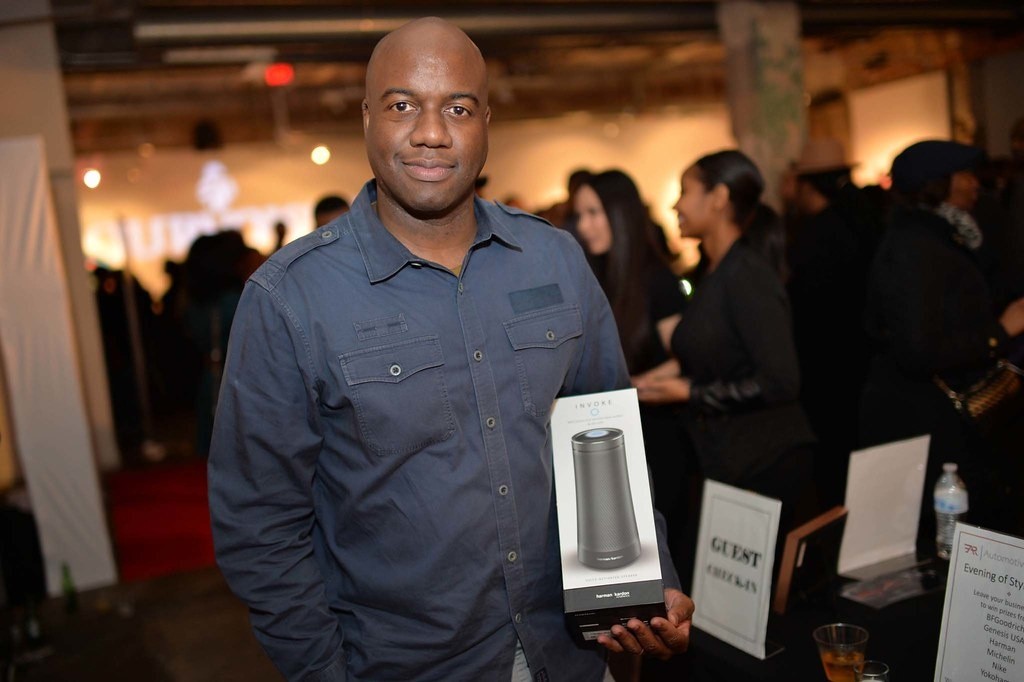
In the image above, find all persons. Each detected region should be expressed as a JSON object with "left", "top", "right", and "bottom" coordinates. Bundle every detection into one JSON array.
[
  {"left": 788, "top": 122, "right": 1024, "bottom": 489},
  {"left": 210, "top": 17, "right": 697, "bottom": 682},
  {"left": 92, "top": 191, "right": 351, "bottom": 473},
  {"left": 560, "top": 164, "right": 687, "bottom": 566},
  {"left": 634, "top": 145, "right": 821, "bottom": 587}
]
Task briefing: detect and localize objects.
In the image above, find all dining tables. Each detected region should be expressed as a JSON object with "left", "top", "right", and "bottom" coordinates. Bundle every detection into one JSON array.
[{"left": 641, "top": 495, "right": 1024, "bottom": 682}]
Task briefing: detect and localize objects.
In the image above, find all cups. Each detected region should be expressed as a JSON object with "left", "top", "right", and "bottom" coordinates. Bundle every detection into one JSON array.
[
  {"left": 852, "top": 660, "right": 890, "bottom": 682},
  {"left": 814, "top": 623, "right": 869, "bottom": 682}
]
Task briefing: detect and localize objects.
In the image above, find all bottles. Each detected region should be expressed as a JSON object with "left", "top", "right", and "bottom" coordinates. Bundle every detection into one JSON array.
[{"left": 935, "top": 461, "right": 969, "bottom": 561}]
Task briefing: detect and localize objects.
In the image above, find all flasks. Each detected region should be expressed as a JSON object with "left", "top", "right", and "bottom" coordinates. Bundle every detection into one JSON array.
[{"left": 572, "top": 427, "right": 643, "bottom": 571}]
